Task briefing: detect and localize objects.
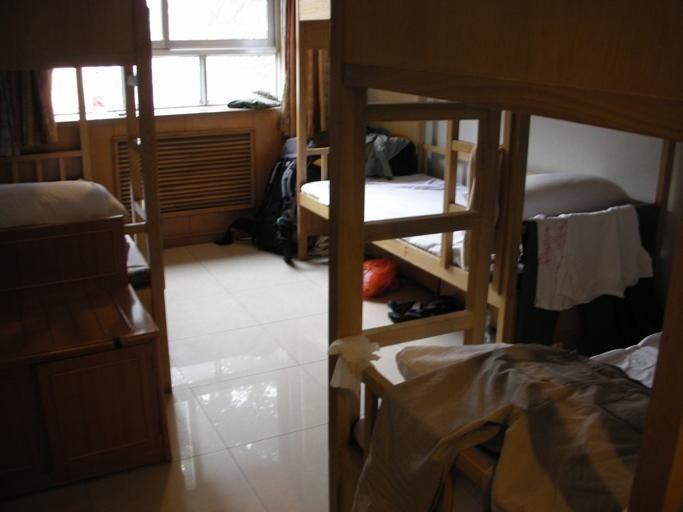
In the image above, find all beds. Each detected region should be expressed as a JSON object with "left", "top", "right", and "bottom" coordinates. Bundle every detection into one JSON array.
[
  {"left": 329, "top": 1, "right": 683, "bottom": 510},
  {"left": 1, "top": 0, "right": 177, "bottom": 490},
  {"left": 295, "top": 0, "right": 675, "bottom": 343}
]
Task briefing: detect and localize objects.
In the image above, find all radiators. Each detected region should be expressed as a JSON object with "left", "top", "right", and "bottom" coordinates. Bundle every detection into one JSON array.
[{"left": 111, "top": 125, "right": 257, "bottom": 223}]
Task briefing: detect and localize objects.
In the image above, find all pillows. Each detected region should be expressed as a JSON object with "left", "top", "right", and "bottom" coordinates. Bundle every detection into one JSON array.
[
  {"left": 460, "top": 170, "right": 630, "bottom": 224},
  {"left": 0, "top": 178, "right": 129, "bottom": 228},
  {"left": 395, "top": 342, "right": 517, "bottom": 380}
]
